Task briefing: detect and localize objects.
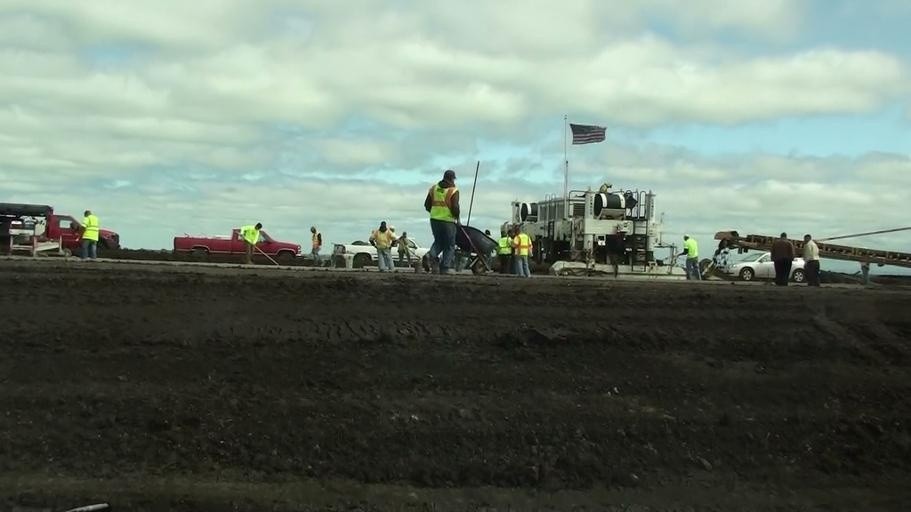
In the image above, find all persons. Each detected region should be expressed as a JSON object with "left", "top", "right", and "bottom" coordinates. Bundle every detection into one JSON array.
[
  {"left": 771, "top": 232, "right": 795, "bottom": 286},
  {"left": 396, "top": 231, "right": 417, "bottom": 266},
  {"left": 78, "top": 210, "right": 99, "bottom": 258},
  {"left": 369, "top": 221, "right": 397, "bottom": 271},
  {"left": 240, "top": 222, "right": 263, "bottom": 265},
  {"left": 423, "top": 170, "right": 462, "bottom": 274},
  {"left": 389, "top": 226, "right": 395, "bottom": 232},
  {"left": 802, "top": 234, "right": 820, "bottom": 286},
  {"left": 484, "top": 228, "right": 533, "bottom": 276},
  {"left": 682, "top": 234, "right": 702, "bottom": 280},
  {"left": 310, "top": 226, "right": 322, "bottom": 265}
]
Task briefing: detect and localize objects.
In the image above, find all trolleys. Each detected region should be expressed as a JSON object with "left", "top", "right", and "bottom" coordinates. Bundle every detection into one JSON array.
[{"left": 449, "top": 218, "right": 499, "bottom": 274}]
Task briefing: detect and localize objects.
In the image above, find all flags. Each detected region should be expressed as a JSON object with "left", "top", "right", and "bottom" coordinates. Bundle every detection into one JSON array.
[{"left": 570, "top": 123, "right": 607, "bottom": 145}]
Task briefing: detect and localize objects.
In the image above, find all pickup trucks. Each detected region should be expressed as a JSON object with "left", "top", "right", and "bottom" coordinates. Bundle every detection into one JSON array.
[
  {"left": 172, "top": 226, "right": 302, "bottom": 257},
  {"left": 331, "top": 234, "right": 437, "bottom": 272}
]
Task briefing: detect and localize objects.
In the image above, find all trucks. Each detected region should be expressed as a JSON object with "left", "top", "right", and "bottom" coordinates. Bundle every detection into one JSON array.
[{"left": 0, "top": 200, "right": 125, "bottom": 250}]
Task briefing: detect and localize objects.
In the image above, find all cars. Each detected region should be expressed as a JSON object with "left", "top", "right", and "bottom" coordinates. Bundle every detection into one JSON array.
[{"left": 722, "top": 251, "right": 807, "bottom": 284}]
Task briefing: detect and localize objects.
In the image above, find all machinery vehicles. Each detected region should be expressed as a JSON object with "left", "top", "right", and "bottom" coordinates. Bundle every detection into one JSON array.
[{"left": 497, "top": 186, "right": 688, "bottom": 280}]
